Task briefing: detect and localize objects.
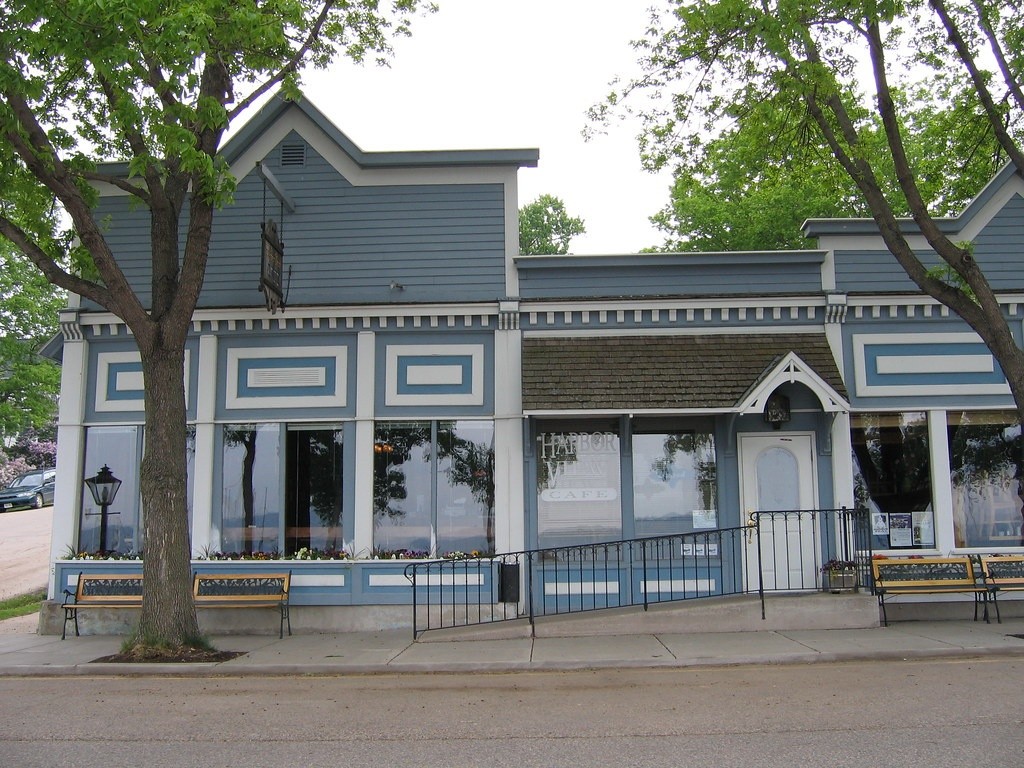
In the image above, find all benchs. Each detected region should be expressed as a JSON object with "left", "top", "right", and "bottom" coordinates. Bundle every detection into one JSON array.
[
  {"left": 61, "top": 570, "right": 293, "bottom": 641},
  {"left": 872, "top": 556, "right": 1024, "bottom": 628}
]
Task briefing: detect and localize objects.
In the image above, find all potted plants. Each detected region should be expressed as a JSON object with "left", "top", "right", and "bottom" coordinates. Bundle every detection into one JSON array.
[{"left": 819, "top": 557, "right": 860, "bottom": 594}]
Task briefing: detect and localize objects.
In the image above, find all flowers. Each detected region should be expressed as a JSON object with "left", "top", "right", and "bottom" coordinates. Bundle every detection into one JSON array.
[
  {"left": 195, "top": 540, "right": 366, "bottom": 559},
  {"left": 368, "top": 544, "right": 499, "bottom": 561}
]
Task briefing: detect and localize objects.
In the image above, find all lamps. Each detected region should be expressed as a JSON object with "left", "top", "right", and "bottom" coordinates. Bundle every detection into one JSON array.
[{"left": 83, "top": 464, "right": 122, "bottom": 505}]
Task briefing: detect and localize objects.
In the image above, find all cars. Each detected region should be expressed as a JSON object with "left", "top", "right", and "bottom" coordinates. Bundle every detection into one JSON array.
[{"left": 0, "top": 468, "right": 56, "bottom": 512}]
